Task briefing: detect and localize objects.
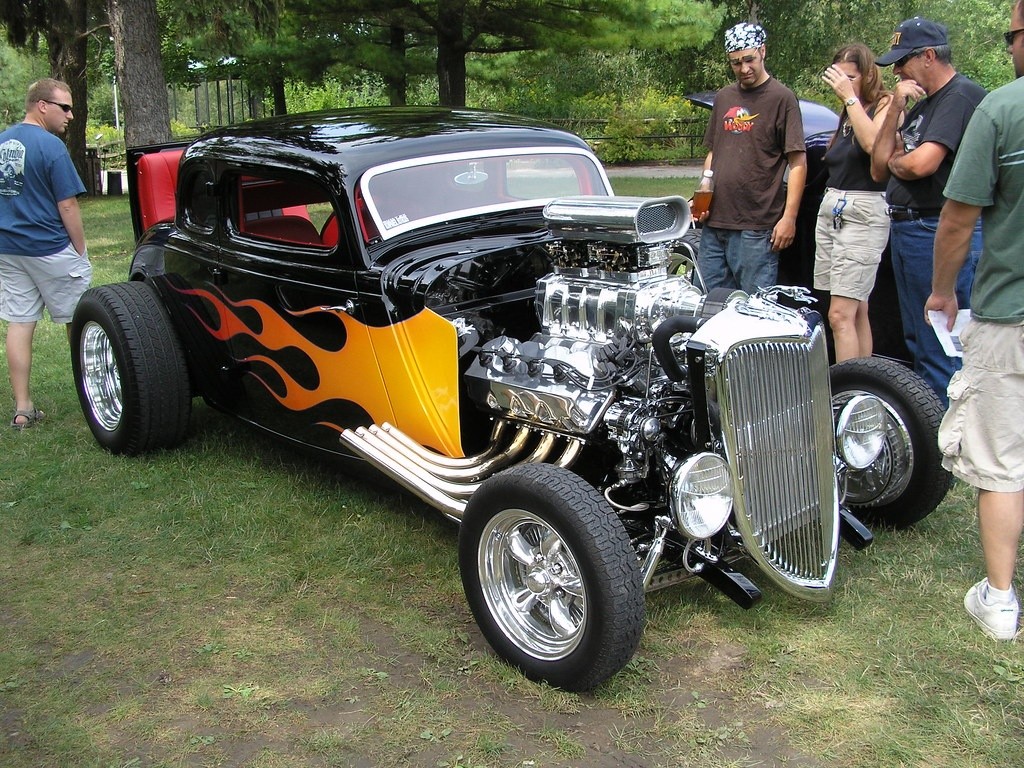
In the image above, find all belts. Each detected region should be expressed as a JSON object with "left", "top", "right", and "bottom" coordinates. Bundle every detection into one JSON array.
[{"left": 887, "top": 204, "right": 947, "bottom": 221}]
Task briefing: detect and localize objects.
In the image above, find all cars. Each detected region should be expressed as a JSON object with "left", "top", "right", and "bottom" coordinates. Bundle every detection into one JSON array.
[
  {"left": 66, "top": 103, "right": 961, "bottom": 694},
  {"left": 682, "top": 90, "right": 846, "bottom": 177}
]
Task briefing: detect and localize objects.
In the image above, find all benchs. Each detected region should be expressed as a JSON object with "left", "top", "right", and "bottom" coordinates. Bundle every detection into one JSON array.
[{"left": 135, "top": 150, "right": 314, "bottom": 232}]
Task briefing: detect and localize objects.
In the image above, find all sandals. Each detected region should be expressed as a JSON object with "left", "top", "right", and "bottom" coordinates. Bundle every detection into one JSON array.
[{"left": 10, "top": 407, "right": 44, "bottom": 428}]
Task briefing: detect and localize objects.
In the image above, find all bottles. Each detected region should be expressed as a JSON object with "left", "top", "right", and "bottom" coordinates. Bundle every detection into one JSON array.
[{"left": 693, "top": 170, "right": 714, "bottom": 218}]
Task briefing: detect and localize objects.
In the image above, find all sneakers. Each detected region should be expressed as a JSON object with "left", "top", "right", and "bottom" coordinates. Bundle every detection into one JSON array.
[{"left": 964, "top": 575, "right": 1018, "bottom": 641}]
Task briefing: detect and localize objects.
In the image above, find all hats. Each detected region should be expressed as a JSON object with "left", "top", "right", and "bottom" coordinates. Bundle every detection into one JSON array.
[{"left": 873, "top": 19, "right": 947, "bottom": 66}]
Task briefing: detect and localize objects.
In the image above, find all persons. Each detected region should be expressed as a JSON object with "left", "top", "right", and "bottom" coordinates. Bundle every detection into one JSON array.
[
  {"left": 924, "top": 0, "right": 1023, "bottom": 637},
  {"left": 0, "top": 78, "right": 94, "bottom": 429},
  {"left": 812, "top": 45, "right": 905, "bottom": 363},
  {"left": 693, "top": 23, "right": 808, "bottom": 298},
  {"left": 871, "top": 14, "right": 987, "bottom": 453}
]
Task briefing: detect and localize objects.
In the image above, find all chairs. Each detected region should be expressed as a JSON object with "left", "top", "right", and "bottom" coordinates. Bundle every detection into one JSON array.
[{"left": 321, "top": 196, "right": 382, "bottom": 248}]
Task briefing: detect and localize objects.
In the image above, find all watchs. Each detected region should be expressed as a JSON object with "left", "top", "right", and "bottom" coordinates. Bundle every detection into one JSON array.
[{"left": 846, "top": 97, "right": 857, "bottom": 106}]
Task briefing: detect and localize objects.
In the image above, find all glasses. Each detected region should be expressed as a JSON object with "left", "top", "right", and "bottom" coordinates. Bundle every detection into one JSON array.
[
  {"left": 848, "top": 73, "right": 864, "bottom": 85},
  {"left": 1003, "top": 28, "right": 1024, "bottom": 45},
  {"left": 896, "top": 48, "right": 939, "bottom": 67},
  {"left": 37, "top": 98, "right": 74, "bottom": 112}
]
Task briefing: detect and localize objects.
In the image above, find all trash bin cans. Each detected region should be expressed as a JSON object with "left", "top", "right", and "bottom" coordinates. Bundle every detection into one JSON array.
[
  {"left": 86, "top": 148, "right": 103, "bottom": 196},
  {"left": 108, "top": 171, "right": 122, "bottom": 195}
]
